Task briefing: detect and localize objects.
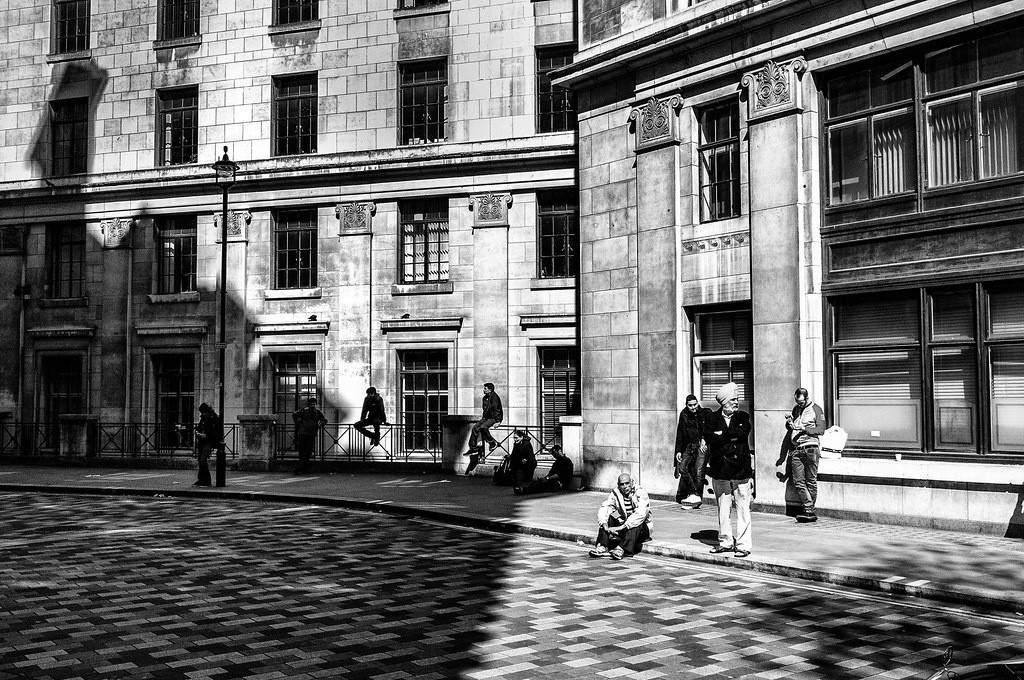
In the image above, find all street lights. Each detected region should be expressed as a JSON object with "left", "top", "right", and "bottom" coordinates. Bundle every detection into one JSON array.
[{"left": 212, "top": 145, "right": 241, "bottom": 486}]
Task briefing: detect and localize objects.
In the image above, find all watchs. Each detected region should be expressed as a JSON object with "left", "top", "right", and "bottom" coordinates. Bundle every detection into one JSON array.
[{"left": 545, "top": 476, "right": 550, "bottom": 480}]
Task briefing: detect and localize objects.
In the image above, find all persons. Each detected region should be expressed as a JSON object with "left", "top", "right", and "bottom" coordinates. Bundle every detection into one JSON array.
[
  {"left": 463, "top": 383, "right": 504, "bottom": 456},
  {"left": 292, "top": 398, "right": 328, "bottom": 474},
  {"left": 703, "top": 382, "right": 753, "bottom": 558},
  {"left": 353, "top": 387, "right": 386, "bottom": 446},
  {"left": 675, "top": 394, "right": 710, "bottom": 510},
  {"left": 785, "top": 388, "right": 826, "bottom": 521},
  {"left": 192, "top": 403, "right": 218, "bottom": 489},
  {"left": 506, "top": 430, "right": 537, "bottom": 481},
  {"left": 513, "top": 444, "right": 574, "bottom": 496},
  {"left": 589, "top": 473, "right": 653, "bottom": 560}
]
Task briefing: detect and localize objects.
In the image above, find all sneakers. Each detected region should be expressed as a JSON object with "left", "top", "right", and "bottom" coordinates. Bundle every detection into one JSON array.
[
  {"left": 590, "top": 547, "right": 612, "bottom": 557},
  {"left": 463, "top": 450, "right": 478, "bottom": 456},
  {"left": 489, "top": 441, "right": 497, "bottom": 451},
  {"left": 681, "top": 495, "right": 702, "bottom": 506},
  {"left": 681, "top": 504, "right": 697, "bottom": 509},
  {"left": 610, "top": 548, "right": 625, "bottom": 559}
]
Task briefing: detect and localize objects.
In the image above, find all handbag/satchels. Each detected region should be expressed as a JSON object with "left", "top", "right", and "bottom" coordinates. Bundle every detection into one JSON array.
[
  {"left": 817, "top": 426, "right": 848, "bottom": 459},
  {"left": 494, "top": 455, "right": 512, "bottom": 484}
]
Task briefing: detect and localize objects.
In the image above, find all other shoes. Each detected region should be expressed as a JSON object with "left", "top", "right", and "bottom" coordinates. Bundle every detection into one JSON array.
[{"left": 796, "top": 512, "right": 818, "bottom": 522}]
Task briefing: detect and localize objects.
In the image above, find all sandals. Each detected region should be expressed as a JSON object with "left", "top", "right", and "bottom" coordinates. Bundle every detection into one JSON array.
[
  {"left": 734, "top": 550, "right": 751, "bottom": 557},
  {"left": 710, "top": 545, "right": 735, "bottom": 553}
]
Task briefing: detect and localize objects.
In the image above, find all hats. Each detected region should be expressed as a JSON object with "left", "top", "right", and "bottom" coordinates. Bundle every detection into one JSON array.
[{"left": 716, "top": 383, "right": 739, "bottom": 407}]
